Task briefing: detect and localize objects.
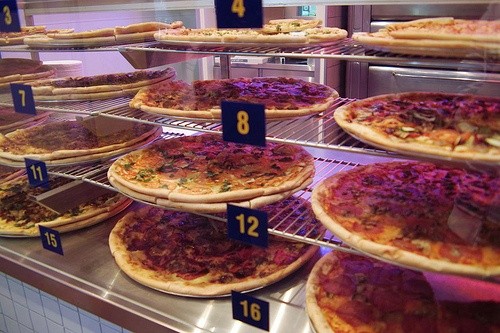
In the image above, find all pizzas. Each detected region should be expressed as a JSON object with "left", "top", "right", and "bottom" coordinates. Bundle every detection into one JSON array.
[{"left": 0, "top": 18, "right": 500, "bottom": 333}]
